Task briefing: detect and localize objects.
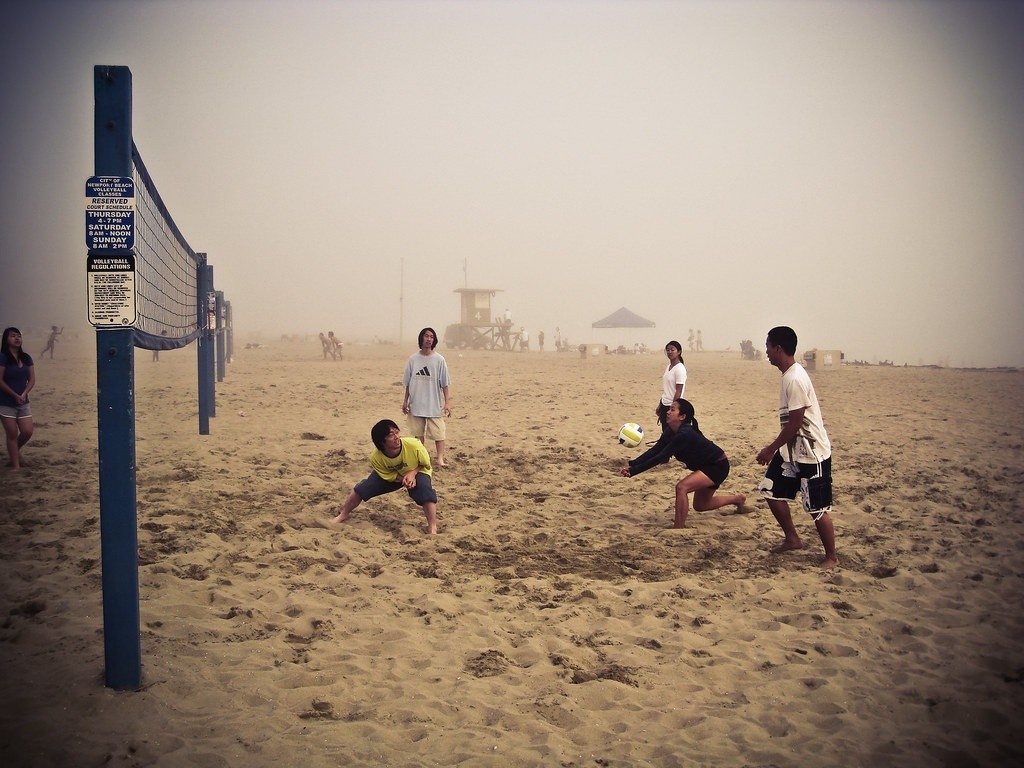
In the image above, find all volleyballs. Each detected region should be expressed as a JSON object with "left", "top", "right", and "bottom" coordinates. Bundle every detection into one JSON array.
[{"left": 618, "top": 422, "right": 644, "bottom": 448}]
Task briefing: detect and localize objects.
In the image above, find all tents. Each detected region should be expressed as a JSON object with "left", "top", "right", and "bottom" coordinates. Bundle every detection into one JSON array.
[{"left": 591, "top": 307, "right": 656, "bottom": 354}]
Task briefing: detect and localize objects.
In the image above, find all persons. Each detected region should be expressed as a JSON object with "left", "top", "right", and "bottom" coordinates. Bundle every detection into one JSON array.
[
  {"left": 37, "top": 326, "right": 64, "bottom": 359},
  {"left": 0, "top": 327, "right": 36, "bottom": 469},
  {"left": 753, "top": 325, "right": 839, "bottom": 570},
  {"left": 151, "top": 329, "right": 167, "bottom": 361},
  {"left": 502, "top": 307, "right": 647, "bottom": 356},
  {"left": 687, "top": 328, "right": 694, "bottom": 353},
  {"left": 328, "top": 331, "right": 343, "bottom": 360},
  {"left": 328, "top": 419, "right": 438, "bottom": 535},
  {"left": 618, "top": 398, "right": 746, "bottom": 529},
  {"left": 696, "top": 330, "right": 704, "bottom": 352},
  {"left": 654, "top": 340, "right": 688, "bottom": 434},
  {"left": 400, "top": 327, "right": 452, "bottom": 466},
  {"left": 739, "top": 339, "right": 762, "bottom": 361},
  {"left": 319, "top": 333, "right": 334, "bottom": 359}
]
